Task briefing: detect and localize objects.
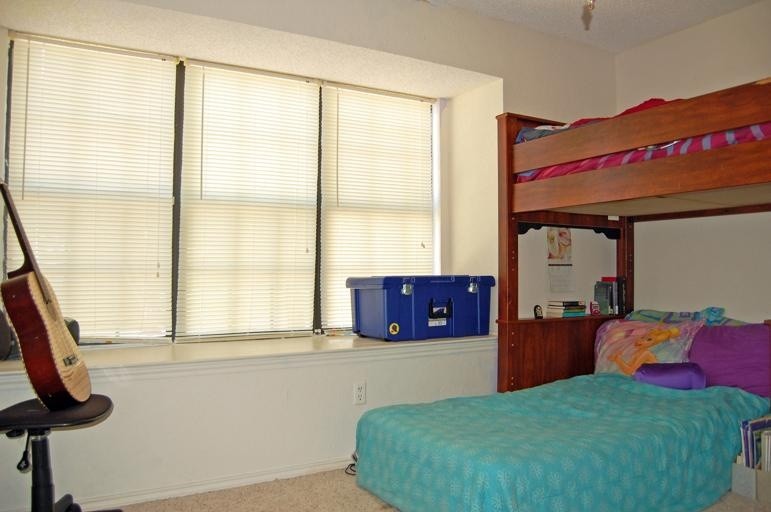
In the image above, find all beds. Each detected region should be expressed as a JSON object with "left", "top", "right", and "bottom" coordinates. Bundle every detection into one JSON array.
[{"left": 350, "top": 74, "right": 771, "bottom": 512}]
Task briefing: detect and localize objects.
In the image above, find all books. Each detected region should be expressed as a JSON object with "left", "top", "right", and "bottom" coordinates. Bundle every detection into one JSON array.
[
  {"left": 735, "top": 414, "right": 771, "bottom": 474},
  {"left": 547, "top": 276, "right": 627, "bottom": 319}
]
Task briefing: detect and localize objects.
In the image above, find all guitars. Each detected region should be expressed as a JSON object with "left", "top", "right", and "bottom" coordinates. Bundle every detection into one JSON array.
[{"left": 0, "top": 181, "right": 92, "bottom": 409}]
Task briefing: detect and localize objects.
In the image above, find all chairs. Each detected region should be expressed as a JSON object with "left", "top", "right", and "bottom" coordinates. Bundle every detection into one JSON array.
[{"left": 1, "top": 310, "right": 124, "bottom": 512}]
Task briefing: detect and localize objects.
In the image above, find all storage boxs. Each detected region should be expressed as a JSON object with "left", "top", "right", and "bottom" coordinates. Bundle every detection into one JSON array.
[{"left": 345, "top": 275, "right": 497, "bottom": 343}]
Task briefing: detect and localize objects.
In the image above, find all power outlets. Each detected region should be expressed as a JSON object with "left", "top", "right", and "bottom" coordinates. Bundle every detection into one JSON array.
[{"left": 352, "top": 382, "right": 367, "bottom": 404}]
